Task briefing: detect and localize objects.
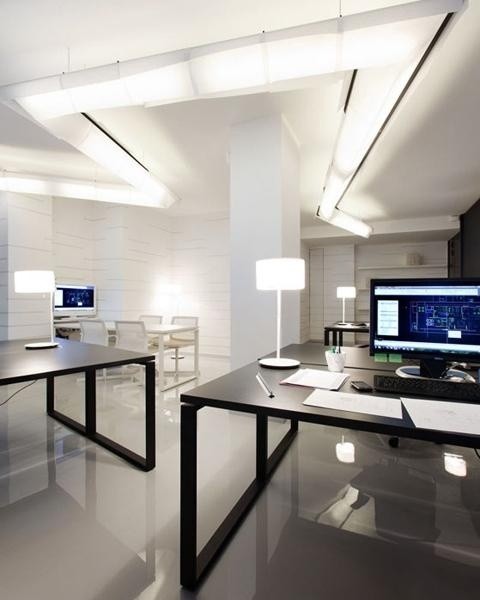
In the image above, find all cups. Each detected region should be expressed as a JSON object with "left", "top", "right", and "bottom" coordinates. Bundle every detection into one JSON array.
[{"left": 324, "top": 350, "right": 347, "bottom": 373}]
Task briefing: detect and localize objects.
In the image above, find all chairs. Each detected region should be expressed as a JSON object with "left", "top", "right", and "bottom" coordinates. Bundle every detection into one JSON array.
[
  {"left": 114, "top": 321, "right": 159, "bottom": 392},
  {"left": 138, "top": 315, "right": 163, "bottom": 348},
  {"left": 77, "top": 318, "right": 124, "bottom": 380},
  {"left": 163, "top": 316, "right": 198, "bottom": 380}
]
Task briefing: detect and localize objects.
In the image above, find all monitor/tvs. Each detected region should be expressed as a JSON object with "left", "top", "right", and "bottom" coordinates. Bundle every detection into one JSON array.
[
  {"left": 52, "top": 285, "right": 96, "bottom": 320},
  {"left": 370, "top": 278, "right": 480, "bottom": 384}
]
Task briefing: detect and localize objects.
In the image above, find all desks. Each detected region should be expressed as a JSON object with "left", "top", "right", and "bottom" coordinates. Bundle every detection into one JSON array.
[
  {"left": 179, "top": 344, "right": 480, "bottom": 589},
  {"left": 52, "top": 323, "right": 199, "bottom": 392},
  {"left": 0, "top": 338, "right": 155, "bottom": 472},
  {"left": 324, "top": 321, "right": 370, "bottom": 346}
]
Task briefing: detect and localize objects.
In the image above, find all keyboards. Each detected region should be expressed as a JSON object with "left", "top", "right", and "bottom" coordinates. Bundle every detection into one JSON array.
[{"left": 374, "top": 375, "right": 480, "bottom": 403}]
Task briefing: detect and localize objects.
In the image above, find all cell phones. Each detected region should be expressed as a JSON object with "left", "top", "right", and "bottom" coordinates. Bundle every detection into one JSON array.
[{"left": 351, "top": 380, "right": 372, "bottom": 391}]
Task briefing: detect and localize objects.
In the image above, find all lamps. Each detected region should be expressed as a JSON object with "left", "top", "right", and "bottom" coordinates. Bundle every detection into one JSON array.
[
  {"left": 255, "top": 258, "right": 305, "bottom": 367},
  {"left": 15, "top": 270, "right": 59, "bottom": 349},
  {"left": 336, "top": 286, "right": 356, "bottom": 325}
]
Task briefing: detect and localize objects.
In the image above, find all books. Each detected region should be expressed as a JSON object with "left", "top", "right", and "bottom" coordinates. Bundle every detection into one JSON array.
[{"left": 278, "top": 367, "right": 351, "bottom": 391}]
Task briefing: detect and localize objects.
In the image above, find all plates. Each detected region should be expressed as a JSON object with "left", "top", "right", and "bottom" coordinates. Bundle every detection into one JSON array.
[
  {"left": 25, "top": 341, "right": 58, "bottom": 349},
  {"left": 258, "top": 358, "right": 299, "bottom": 369}
]
